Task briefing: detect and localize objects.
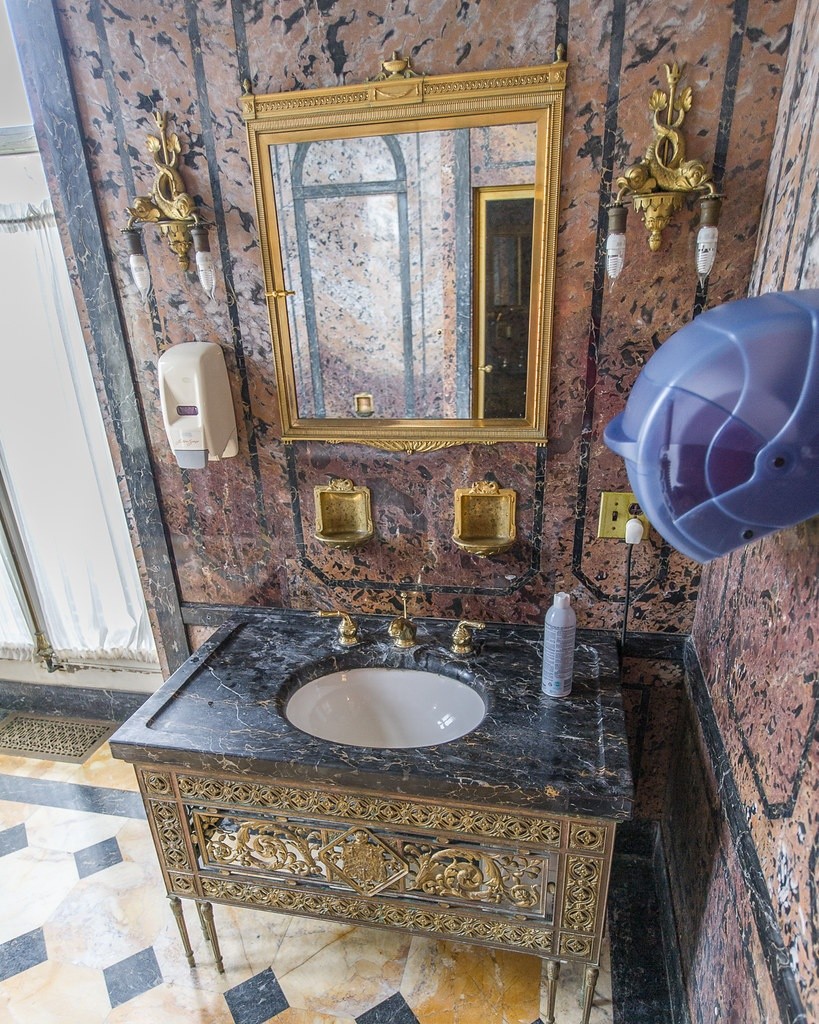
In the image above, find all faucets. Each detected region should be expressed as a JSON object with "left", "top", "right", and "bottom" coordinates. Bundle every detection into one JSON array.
[{"left": 387, "top": 616, "right": 419, "bottom": 650}]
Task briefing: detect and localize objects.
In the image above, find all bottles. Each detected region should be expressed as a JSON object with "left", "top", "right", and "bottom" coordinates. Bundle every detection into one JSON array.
[{"left": 541, "top": 591, "right": 576, "bottom": 697}]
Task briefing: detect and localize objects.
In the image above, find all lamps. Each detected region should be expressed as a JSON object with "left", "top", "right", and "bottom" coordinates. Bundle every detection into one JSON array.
[
  {"left": 604, "top": 60, "right": 727, "bottom": 293},
  {"left": 122, "top": 109, "right": 214, "bottom": 301}
]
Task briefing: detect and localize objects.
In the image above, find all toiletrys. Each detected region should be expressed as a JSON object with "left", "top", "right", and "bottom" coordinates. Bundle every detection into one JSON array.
[{"left": 157, "top": 342, "right": 240, "bottom": 471}]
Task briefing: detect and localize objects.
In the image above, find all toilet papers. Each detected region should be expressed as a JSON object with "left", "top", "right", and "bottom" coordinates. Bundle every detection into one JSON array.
[{"left": 660, "top": 373, "right": 800, "bottom": 497}]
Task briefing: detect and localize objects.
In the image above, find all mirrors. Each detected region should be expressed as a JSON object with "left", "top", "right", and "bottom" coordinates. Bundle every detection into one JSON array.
[{"left": 238, "top": 42, "right": 571, "bottom": 453}]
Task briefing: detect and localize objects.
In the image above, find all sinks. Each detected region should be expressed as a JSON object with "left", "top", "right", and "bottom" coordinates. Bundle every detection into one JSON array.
[{"left": 285, "top": 666, "right": 486, "bottom": 748}]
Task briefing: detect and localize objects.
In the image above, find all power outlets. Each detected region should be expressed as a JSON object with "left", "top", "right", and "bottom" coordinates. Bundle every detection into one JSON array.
[{"left": 599, "top": 492, "right": 650, "bottom": 540}]
